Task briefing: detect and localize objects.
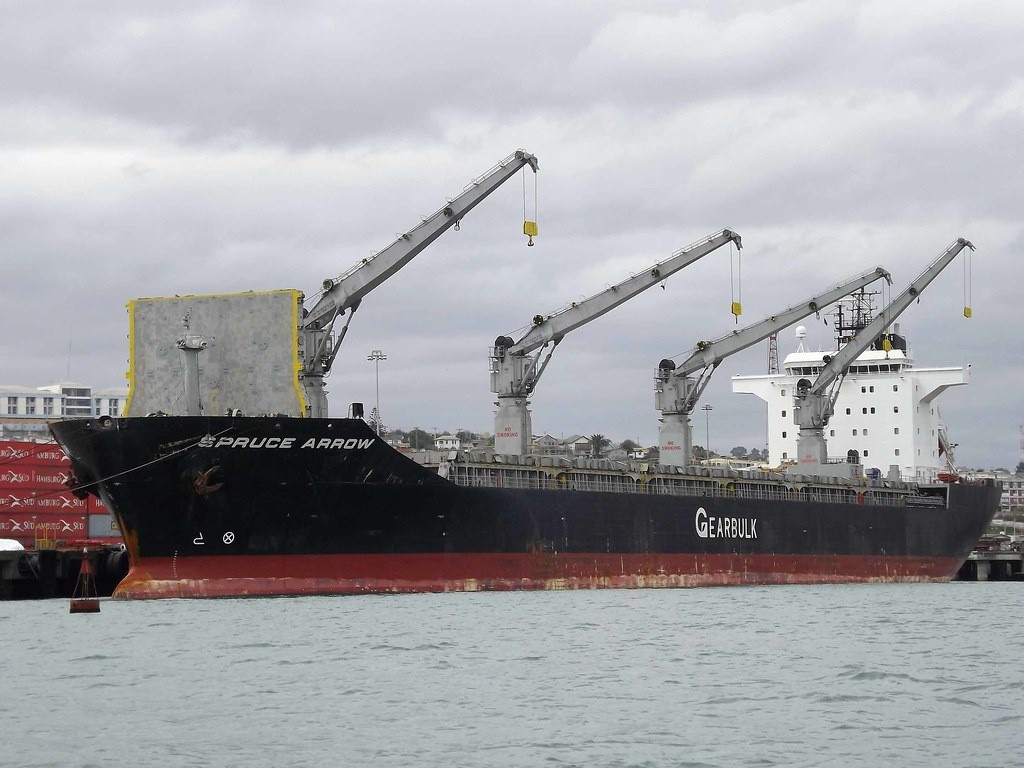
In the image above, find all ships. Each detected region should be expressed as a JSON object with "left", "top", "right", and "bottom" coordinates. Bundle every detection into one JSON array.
[{"left": 48, "top": 415, "right": 1003, "bottom": 602}]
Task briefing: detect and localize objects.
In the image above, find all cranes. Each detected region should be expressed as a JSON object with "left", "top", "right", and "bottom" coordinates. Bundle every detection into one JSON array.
[
  {"left": 792, "top": 238, "right": 977, "bottom": 464},
  {"left": 303, "top": 148, "right": 539, "bottom": 418},
  {"left": 488, "top": 227, "right": 742, "bottom": 456},
  {"left": 653, "top": 265, "right": 894, "bottom": 464}
]
